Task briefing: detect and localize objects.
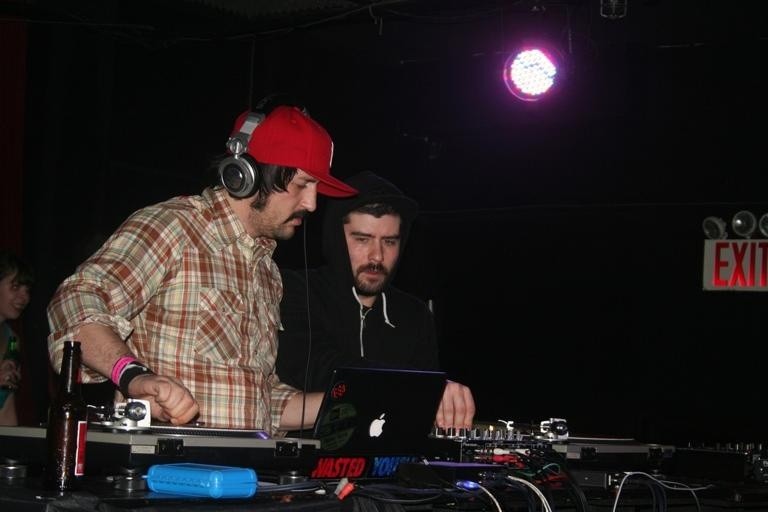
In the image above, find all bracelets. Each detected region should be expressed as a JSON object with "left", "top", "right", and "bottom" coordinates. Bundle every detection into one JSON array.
[
  {"left": 120, "top": 367, "right": 157, "bottom": 399},
  {"left": 111, "top": 356, "right": 140, "bottom": 386}
]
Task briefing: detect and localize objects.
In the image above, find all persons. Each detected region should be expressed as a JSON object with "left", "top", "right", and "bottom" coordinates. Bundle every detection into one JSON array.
[
  {"left": 45, "top": 94, "right": 360, "bottom": 437},
  {"left": 276, "top": 170, "right": 476, "bottom": 438},
  {"left": 0, "top": 247, "right": 34, "bottom": 426}
]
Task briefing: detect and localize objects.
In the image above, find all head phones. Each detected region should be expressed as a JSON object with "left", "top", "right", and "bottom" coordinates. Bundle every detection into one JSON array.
[{"left": 219, "top": 94, "right": 280, "bottom": 199}]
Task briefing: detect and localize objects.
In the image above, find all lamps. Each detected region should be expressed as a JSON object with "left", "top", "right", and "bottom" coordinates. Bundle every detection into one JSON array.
[{"left": 703, "top": 211, "right": 768, "bottom": 241}]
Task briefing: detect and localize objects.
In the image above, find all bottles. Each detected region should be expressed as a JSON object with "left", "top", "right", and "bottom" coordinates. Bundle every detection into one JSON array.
[
  {"left": 4, "top": 335, "right": 21, "bottom": 390},
  {"left": 43, "top": 341, "right": 89, "bottom": 492}
]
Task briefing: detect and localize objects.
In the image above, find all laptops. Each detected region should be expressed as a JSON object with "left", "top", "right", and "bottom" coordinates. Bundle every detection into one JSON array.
[{"left": 279, "top": 367, "right": 447, "bottom": 452}]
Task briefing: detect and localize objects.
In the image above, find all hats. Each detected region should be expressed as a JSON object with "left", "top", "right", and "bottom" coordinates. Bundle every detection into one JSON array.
[{"left": 227, "top": 105, "right": 359, "bottom": 197}]
